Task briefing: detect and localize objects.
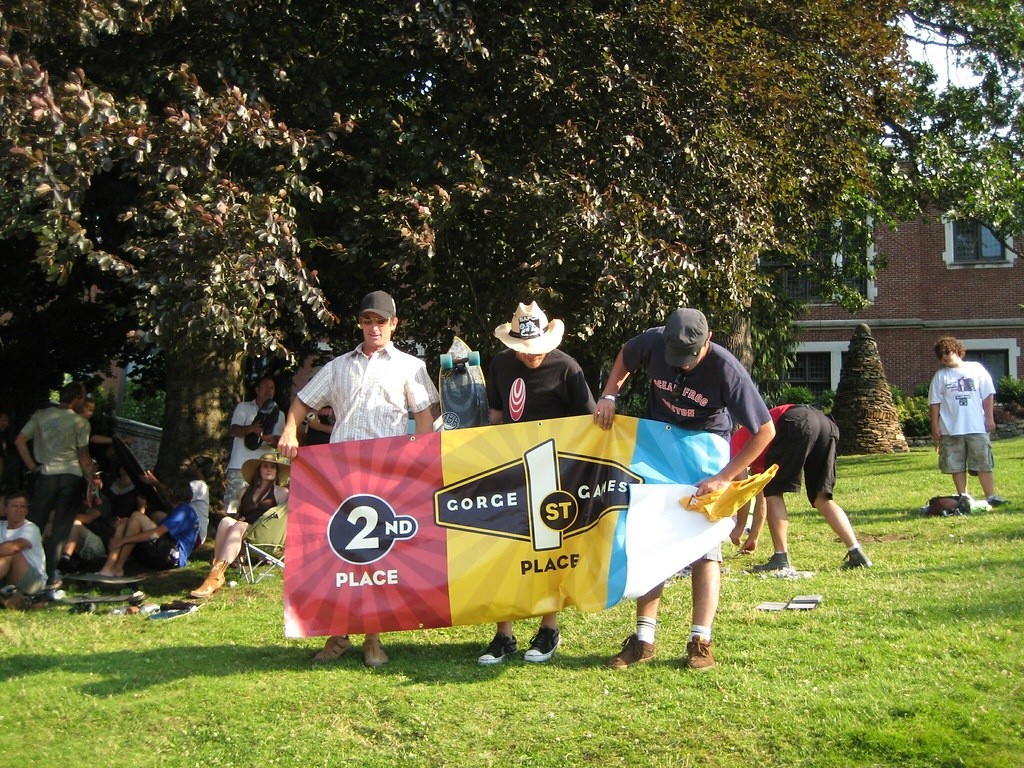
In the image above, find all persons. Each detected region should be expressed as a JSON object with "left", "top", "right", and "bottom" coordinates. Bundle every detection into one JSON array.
[
  {"left": 224, "top": 375, "right": 286, "bottom": 568},
  {"left": 730, "top": 403, "right": 873, "bottom": 574},
  {"left": 478, "top": 301, "right": 589, "bottom": 664},
  {"left": 297, "top": 404, "right": 334, "bottom": 445},
  {"left": 277, "top": 291, "right": 440, "bottom": 667},
  {"left": 190, "top": 453, "right": 291, "bottom": 598},
  {"left": 0, "top": 381, "right": 213, "bottom": 609},
  {"left": 596, "top": 308, "right": 775, "bottom": 668},
  {"left": 928, "top": 336, "right": 1010, "bottom": 507}
]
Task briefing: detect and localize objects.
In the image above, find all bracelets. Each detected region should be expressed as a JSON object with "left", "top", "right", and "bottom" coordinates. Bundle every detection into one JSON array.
[{"left": 599, "top": 395, "right": 617, "bottom": 403}]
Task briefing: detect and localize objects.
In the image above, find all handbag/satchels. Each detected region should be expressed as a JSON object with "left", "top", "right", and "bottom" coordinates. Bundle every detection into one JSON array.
[
  {"left": 949, "top": 495, "right": 971, "bottom": 514},
  {"left": 929, "top": 495, "right": 958, "bottom": 516}
]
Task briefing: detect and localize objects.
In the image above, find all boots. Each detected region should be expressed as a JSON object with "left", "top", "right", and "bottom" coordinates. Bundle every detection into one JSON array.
[{"left": 190, "top": 560, "right": 227, "bottom": 598}]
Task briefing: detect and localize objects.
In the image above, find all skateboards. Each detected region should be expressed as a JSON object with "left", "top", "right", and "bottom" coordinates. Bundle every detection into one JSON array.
[
  {"left": 65, "top": 592, "right": 146, "bottom": 613},
  {"left": 436, "top": 335, "right": 490, "bottom": 430},
  {"left": 111, "top": 434, "right": 174, "bottom": 515},
  {"left": 64, "top": 567, "right": 150, "bottom": 596},
  {"left": 148, "top": 596, "right": 206, "bottom": 623}
]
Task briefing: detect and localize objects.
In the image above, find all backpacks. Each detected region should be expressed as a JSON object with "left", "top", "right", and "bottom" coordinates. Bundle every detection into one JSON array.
[{"left": 243, "top": 501, "right": 288, "bottom": 562}]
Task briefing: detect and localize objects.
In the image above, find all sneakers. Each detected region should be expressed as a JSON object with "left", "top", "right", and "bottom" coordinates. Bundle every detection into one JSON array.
[
  {"left": 477, "top": 631, "right": 519, "bottom": 667},
  {"left": 524, "top": 625, "right": 561, "bottom": 662},
  {"left": 838, "top": 546, "right": 872, "bottom": 571},
  {"left": 607, "top": 634, "right": 657, "bottom": 670},
  {"left": 753, "top": 552, "right": 790, "bottom": 574},
  {"left": 685, "top": 634, "right": 717, "bottom": 672}
]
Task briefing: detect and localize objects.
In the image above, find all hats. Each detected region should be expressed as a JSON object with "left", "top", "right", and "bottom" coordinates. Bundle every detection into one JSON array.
[
  {"left": 241, "top": 454, "right": 291, "bottom": 485},
  {"left": 661, "top": 307, "right": 709, "bottom": 368},
  {"left": 359, "top": 290, "right": 396, "bottom": 320},
  {"left": 495, "top": 300, "right": 565, "bottom": 354},
  {"left": 310, "top": 354, "right": 336, "bottom": 368},
  {"left": 194, "top": 455, "right": 213, "bottom": 478}
]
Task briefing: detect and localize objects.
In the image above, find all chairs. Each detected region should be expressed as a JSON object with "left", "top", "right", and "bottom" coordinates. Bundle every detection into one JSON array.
[{"left": 237, "top": 502, "right": 288, "bottom": 584}]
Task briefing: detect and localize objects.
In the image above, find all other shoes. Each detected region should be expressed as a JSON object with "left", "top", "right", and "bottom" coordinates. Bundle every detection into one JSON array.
[
  {"left": 990, "top": 497, "right": 1009, "bottom": 509},
  {"left": 313, "top": 634, "right": 354, "bottom": 663},
  {"left": 363, "top": 639, "right": 390, "bottom": 668},
  {"left": 45, "top": 577, "right": 63, "bottom": 589}
]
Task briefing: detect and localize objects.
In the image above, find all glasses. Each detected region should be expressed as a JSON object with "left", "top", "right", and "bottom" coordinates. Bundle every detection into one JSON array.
[
  {"left": 937, "top": 350, "right": 951, "bottom": 359},
  {"left": 359, "top": 315, "right": 390, "bottom": 326}
]
